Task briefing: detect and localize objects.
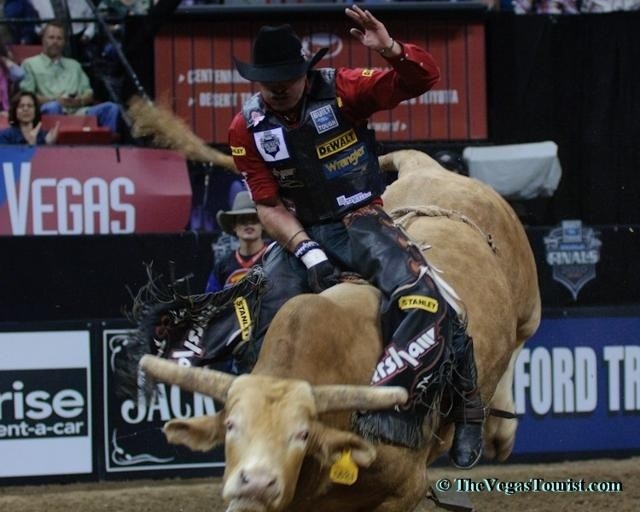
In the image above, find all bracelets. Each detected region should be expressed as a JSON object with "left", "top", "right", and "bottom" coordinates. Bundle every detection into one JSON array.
[{"left": 379, "top": 38, "right": 394, "bottom": 58}]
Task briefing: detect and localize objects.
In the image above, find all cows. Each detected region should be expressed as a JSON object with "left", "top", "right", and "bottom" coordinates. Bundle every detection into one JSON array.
[{"left": 123, "top": 94, "right": 545, "bottom": 512}]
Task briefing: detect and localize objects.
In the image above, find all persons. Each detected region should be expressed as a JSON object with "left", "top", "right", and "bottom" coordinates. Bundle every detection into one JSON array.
[
  {"left": 0, "top": 89, "right": 63, "bottom": 147},
  {"left": 0, "top": 0, "right": 196, "bottom": 121},
  {"left": 17, "top": 21, "right": 120, "bottom": 132},
  {"left": 227, "top": 2, "right": 490, "bottom": 471},
  {"left": 204, "top": 189, "right": 273, "bottom": 298}
]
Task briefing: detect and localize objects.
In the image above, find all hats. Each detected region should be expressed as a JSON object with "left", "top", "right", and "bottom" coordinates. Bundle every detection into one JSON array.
[
  {"left": 216, "top": 191, "right": 257, "bottom": 236},
  {"left": 234, "top": 24, "right": 329, "bottom": 84}
]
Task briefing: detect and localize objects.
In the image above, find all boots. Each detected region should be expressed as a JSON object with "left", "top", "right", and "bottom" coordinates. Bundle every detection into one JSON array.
[{"left": 449, "top": 338, "right": 483, "bottom": 470}]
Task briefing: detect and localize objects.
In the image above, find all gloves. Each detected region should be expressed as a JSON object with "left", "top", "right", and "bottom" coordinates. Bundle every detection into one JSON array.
[{"left": 294, "top": 240, "right": 334, "bottom": 293}]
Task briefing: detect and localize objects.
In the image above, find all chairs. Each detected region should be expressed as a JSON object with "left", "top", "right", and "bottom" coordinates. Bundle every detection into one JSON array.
[{"left": 0, "top": 44, "right": 120, "bottom": 144}]
